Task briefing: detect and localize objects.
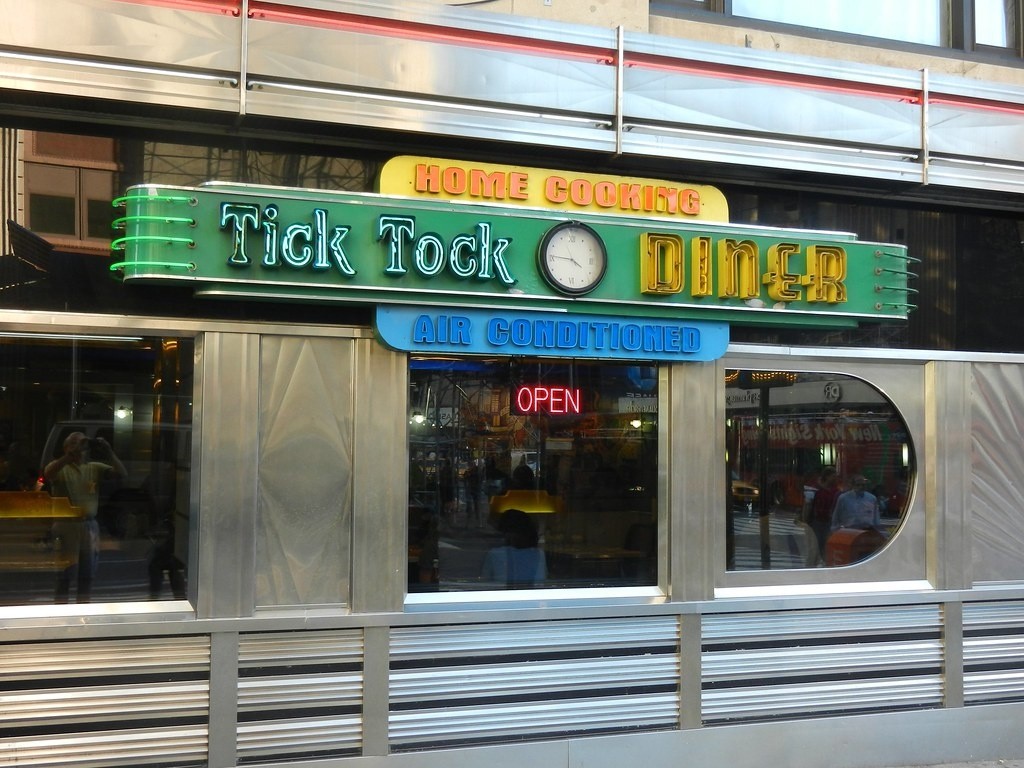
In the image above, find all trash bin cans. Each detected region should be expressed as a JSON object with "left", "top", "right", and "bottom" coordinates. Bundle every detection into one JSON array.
[{"left": 824, "top": 526, "right": 886, "bottom": 568}]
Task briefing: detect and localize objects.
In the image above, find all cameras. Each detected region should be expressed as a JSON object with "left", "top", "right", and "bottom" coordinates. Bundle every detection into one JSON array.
[{"left": 89, "top": 439, "right": 99, "bottom": 448}]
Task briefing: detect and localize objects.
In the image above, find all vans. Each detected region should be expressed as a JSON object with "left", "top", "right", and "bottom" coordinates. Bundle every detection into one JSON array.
[{"left": 36, "top": 419, "right": 192, "bottom": 542}]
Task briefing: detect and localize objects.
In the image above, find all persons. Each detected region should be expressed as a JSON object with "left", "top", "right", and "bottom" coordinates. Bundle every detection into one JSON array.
[{"left": 1, "top": 431, "right": 881, "bottom": 601}]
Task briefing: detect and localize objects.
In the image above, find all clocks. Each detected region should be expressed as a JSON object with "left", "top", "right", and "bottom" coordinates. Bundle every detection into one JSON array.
[{"left": 541, "top": 220, "right": 608, "bottom": 292}]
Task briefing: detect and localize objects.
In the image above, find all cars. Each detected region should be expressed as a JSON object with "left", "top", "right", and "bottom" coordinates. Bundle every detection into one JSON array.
[
  {"left": 731, "top": 479, "right": 760, "bottom": 512},
  {"left": 519, "top": 451, "right": 541, "bottom": 479}
]
